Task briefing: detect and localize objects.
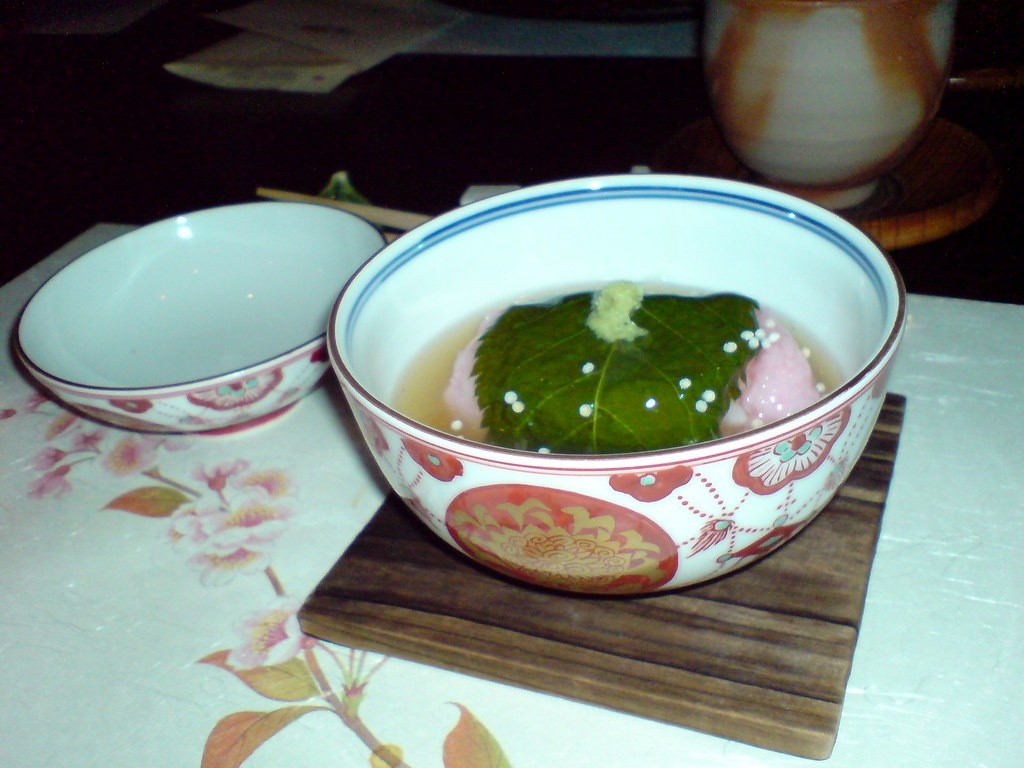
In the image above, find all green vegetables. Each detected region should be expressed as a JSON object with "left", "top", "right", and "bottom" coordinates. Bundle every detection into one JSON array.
[{"left": 468, "top": 285, "right": 763, "bottom": 455}]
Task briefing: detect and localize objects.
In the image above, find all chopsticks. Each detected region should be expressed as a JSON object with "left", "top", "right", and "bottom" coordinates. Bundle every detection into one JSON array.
[{"left": 255, "top": 187, "right": 437, "bottom": 243}]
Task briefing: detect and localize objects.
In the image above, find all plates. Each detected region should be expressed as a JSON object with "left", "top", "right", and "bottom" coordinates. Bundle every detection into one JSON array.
[{"left": 665, "top": 115, "right": 1002, "bottom": 249}]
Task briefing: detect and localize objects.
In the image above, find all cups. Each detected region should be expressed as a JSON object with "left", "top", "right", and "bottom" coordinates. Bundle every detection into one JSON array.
[{"left": 705, "top": 0, "right": 955, "bottom": 211}]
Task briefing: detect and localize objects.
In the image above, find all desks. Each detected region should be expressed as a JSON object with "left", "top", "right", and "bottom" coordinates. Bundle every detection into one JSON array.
[{"left": 0, "top": 222, "right": 1024, "bottom": 768}]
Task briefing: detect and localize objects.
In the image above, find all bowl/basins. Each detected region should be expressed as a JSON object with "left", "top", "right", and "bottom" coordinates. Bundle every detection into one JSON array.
[
  {"left": 13, "top": 201, "right": 390, "bottom": 435},
  {"left": 327, "top": 174, "right": 906, "bottom": 595}
]
